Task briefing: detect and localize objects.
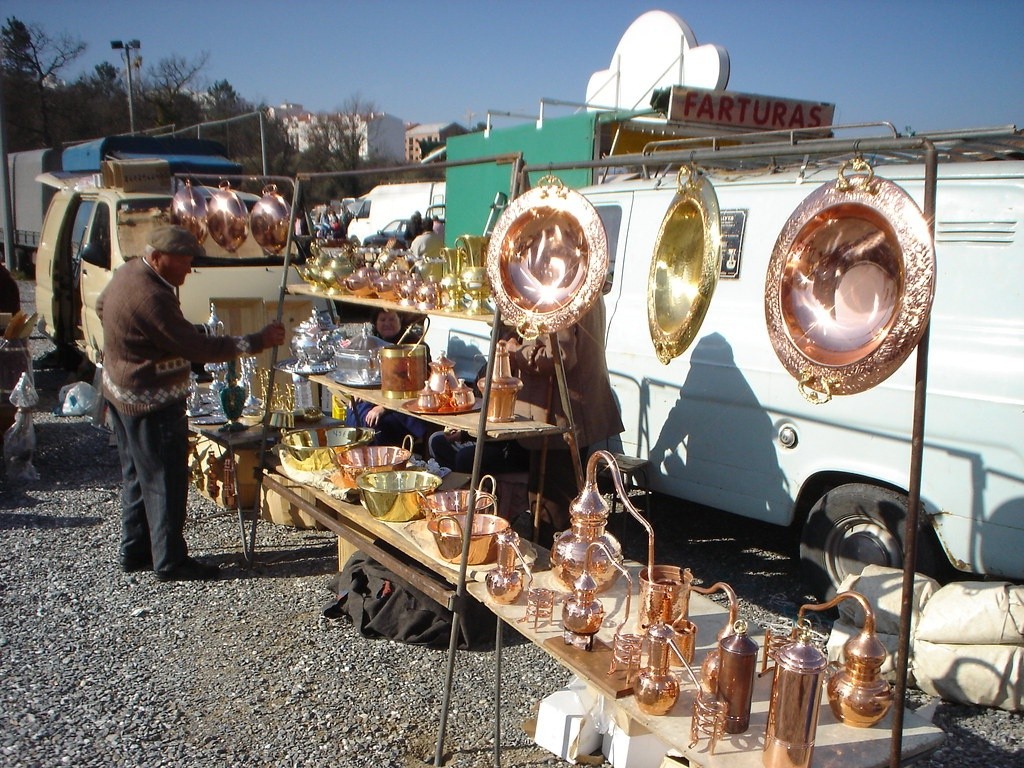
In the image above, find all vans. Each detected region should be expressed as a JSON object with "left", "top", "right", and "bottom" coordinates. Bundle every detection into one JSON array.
[
  {"left": 29, "top": 164, "right": 303, "bottom": 404},
  {"left": 343, "top": 178, "right": 447, "bottom": 246},
  {"left": 571, "top": 117, "right": 1024, "bottom": 612}
]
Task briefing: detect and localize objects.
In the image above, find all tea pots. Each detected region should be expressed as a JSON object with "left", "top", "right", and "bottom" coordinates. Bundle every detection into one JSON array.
[{"left": 291, "top": 234, "right": 499, "bottom": 319}]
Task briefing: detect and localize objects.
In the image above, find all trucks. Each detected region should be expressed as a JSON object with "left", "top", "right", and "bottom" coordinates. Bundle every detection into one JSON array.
[{"left": 0, "top": 133, "right": 247, "bottom": 284}]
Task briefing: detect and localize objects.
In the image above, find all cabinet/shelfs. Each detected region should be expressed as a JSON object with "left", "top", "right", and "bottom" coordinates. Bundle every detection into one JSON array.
[{"left": 186, "top": 279, "right": 945, "bottom": 766}]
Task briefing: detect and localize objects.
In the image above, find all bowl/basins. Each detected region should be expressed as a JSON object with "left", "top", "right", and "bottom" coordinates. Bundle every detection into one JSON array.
[{"left": 281, "top": 422, "right": 516, "bottom": 565}]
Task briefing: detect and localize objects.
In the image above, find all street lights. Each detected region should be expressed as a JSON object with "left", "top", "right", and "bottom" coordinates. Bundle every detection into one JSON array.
[{"left": 110, "top": 37, "right": 141, "bottom": 135}]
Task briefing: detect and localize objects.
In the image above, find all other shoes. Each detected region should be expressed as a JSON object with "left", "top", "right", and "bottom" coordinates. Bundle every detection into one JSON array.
[{"left": 118, "top": 552, "right": 221, "bottom": 581}]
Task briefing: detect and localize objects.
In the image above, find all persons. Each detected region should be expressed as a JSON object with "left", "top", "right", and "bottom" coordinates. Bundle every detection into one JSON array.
[
  {"left": 429, "top": 321, "right": 529, "bottom": 491},
  {"left": 315, "top": 210, "right": 341, "bottom": 238},
  {"left": 404, "top": 211, "right": 444, "bottom": 261},
  {"left": 95, "top": 224, "right": 286, "bottom": 584},
  {"left": 344, "top": 307, "right": 431, "bottom": 456},
  {"left": 507, "top": 270, "right": 624, "bottom": 551}
]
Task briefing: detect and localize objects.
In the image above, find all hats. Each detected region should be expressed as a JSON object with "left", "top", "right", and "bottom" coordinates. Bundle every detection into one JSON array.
[{"left": 147, "top": 225, "right": 206, "bottom": 256}]
